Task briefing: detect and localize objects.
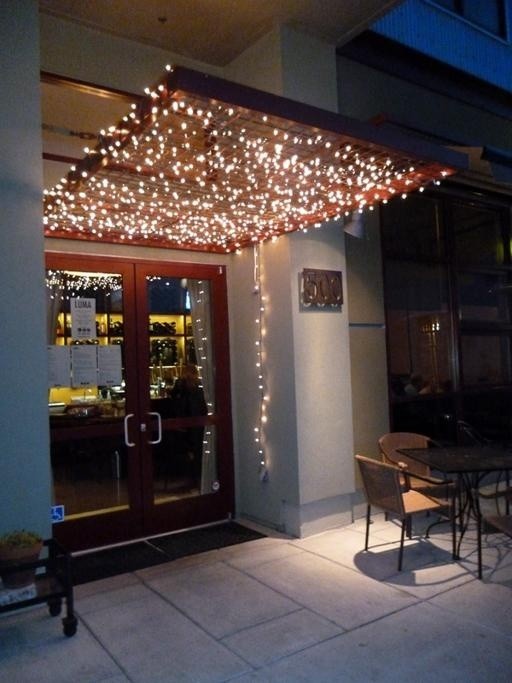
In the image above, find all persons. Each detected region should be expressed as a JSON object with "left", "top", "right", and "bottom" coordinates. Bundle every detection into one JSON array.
[{"left": 170, "top": 363, "right": 208, "bottom": 494}]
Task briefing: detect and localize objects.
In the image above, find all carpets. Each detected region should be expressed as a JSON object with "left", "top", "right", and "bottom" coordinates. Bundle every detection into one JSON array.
[{"left": 75, "top": 521, "right": 267, "bottom": 586}]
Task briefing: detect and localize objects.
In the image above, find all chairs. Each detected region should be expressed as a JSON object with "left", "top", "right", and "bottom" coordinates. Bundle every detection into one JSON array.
[{"left": 350, "top": 420, "right": 510, "bottom": 580}]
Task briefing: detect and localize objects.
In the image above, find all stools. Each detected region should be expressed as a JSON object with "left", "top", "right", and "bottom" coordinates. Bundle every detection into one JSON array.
[{"left": 0, "top": 536, "right": 87, "bottom": 639}]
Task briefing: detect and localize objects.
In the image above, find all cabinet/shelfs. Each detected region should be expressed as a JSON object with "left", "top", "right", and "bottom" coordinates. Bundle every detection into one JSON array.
[{"left": 56, "top": 312, "right": 199, "bottom": 378}]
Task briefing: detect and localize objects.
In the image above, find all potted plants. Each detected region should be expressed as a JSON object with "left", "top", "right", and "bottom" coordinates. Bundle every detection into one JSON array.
[{"left": 0, "top": 529, "right": 44, "bottom": 588}]
